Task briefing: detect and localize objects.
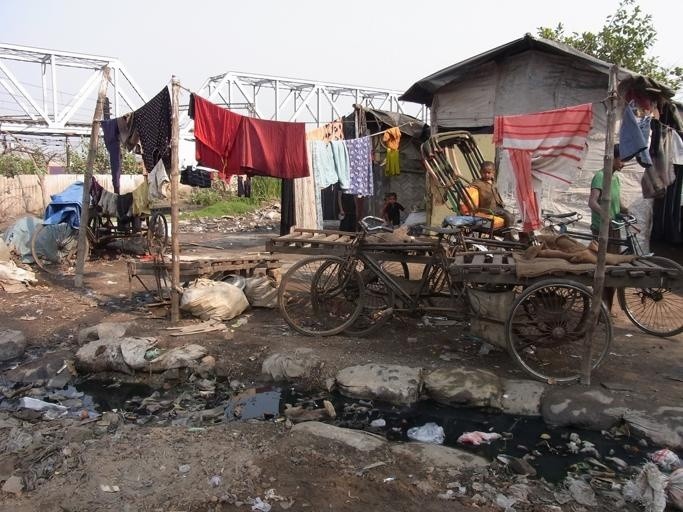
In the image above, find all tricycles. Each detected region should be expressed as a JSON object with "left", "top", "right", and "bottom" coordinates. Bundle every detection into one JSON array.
[{"left": 29, "top": 202, "right": 166, "bottom": 277}]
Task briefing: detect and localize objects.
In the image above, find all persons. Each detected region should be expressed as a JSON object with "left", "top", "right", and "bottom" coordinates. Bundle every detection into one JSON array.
[
  {"left": 588, "top": 144, "right": 629, "bottom": 325},
  {"left": 469, "top": 161, "right": 518, "bottom": 243},
  {"left": 380, "top": 193, "right": 406, "bottom": 226}
]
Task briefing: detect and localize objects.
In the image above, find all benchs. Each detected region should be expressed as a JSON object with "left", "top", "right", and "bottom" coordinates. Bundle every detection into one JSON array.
[{"left": 457, "top": 183, "right": 506, "bottom": 230}]
